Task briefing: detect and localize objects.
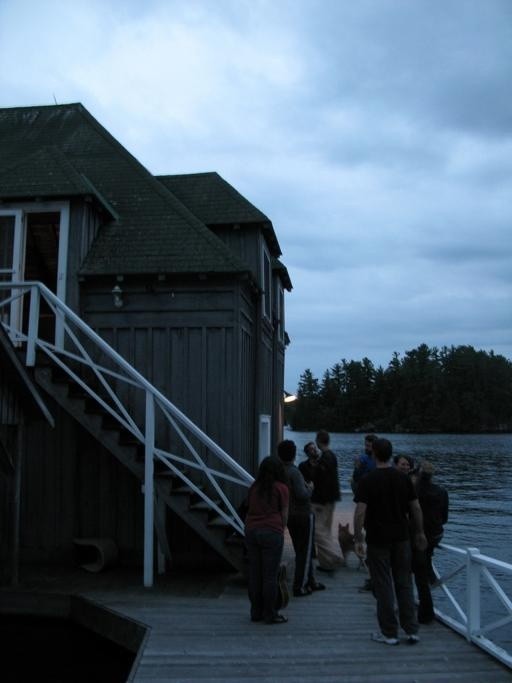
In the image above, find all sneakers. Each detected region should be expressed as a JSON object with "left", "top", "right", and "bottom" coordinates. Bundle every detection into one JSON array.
[
  {"left": 370, "top": 631, "right": 400, "bottom": 644},
  {"left": 407, "top": 634, "right": 420, "bottom": 643}
]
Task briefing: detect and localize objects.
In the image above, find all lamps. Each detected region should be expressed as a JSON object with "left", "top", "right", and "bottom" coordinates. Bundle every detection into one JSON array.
[
  {"left": 112, "top": 285, "right": 123, "bottom": 308},
  {"left": 282, "top": 389, "right": 297, "bottom": 403}
]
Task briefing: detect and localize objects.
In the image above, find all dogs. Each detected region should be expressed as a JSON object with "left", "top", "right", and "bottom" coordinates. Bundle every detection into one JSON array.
[{"left": 338, "top": 523, "right": 370, "bottom": 573}]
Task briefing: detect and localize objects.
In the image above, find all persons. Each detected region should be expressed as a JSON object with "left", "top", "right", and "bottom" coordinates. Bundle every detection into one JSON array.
[
  {"left": 242, "top": 430, "right": 342, "bottom": 624},
  {"left": 351, "top": 436, "right": 451, "bottom": 646}
]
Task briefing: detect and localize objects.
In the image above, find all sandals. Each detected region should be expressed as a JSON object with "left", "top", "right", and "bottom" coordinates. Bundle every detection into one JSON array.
[
  {"left": 311, "top": 582, "right": 325, "bottom": 591},
  {"left": 267, "top": 614, "right": 288, "bottom": 624},
  {"left": 294, "top": 588, "right": 312, "bottom": 596}
]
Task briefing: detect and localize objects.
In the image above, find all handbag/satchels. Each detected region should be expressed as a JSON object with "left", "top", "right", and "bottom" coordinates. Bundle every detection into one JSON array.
[{"left": 276, "top": 560, "right": 290, "bottom": 608}]
